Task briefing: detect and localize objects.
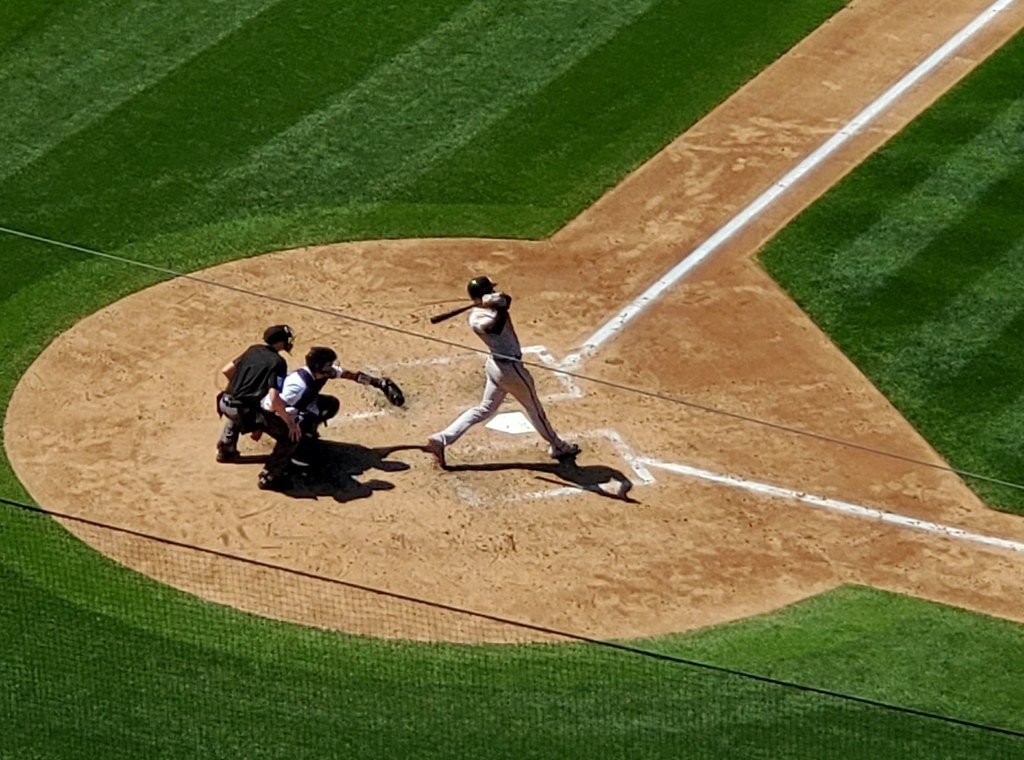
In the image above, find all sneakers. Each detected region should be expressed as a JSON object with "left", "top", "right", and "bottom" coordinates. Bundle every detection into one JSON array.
[
  {"left": 428, "top": 436, "right": 448, "bottom": 468},
  {"left": 548, "top": 441, "right": 579, "bottom": 457}
]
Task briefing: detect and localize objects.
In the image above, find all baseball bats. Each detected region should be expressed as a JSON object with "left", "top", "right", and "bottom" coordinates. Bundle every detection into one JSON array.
[{"left": 432, "top": 300, "right": 483, "bottom": 325}]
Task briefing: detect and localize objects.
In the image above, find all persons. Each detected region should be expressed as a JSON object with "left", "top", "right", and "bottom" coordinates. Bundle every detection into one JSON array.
[
  {"left": 217, "top": 325, "right": 302, "bottom": 488},
  {"left": 264, "top": 345, "right": 405, "bottom": 444},
  {"left": 428, "top": 277, "right": 579, "bottom": 470}
]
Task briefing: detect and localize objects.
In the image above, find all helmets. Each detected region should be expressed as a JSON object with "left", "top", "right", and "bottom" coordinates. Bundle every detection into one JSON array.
[
  {"left": 306, "top": 347, "right": 337, "bottom": 372},
  {"left": 263, "top": 325, "right": 288, "bottom": 343}
]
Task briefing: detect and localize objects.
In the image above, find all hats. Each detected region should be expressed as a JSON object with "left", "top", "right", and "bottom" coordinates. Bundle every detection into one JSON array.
[{"left": 467, "top": 275, "right": 496, "bottom": 300}]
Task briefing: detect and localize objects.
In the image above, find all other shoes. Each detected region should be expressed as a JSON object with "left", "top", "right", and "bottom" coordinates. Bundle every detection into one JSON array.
[
  {"left": 216, "top": 444, "right": 241, "bottom": 462},
  {"left": 259, "top": 471, "right": 292, "bottom": 490}
]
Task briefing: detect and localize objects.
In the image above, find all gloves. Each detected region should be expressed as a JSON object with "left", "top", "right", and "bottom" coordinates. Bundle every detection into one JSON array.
[
  {"left": 481, "top": 292, "right": 500, "bottom": 306},
  {"left": 491, "top": 296, "right": 507, "bottom": 307}
]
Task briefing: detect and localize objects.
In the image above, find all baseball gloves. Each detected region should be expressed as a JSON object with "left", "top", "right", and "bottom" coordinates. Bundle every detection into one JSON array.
[{"left": 373, "top": 376, "right": 405, "bottom": 407}]
